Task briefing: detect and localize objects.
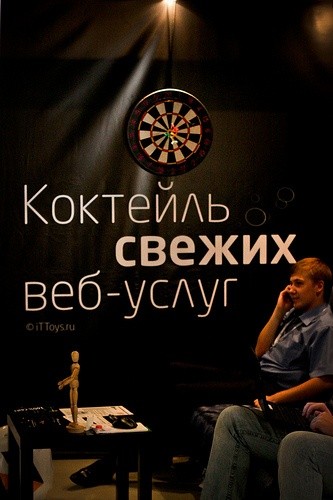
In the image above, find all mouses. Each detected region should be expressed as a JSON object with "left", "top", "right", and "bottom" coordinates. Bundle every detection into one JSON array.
[{"left": 112, "top": 417, "right": 137, "bottom": 428}]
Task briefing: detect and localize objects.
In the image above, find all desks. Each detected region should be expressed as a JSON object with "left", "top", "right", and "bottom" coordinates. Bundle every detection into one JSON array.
[{"left": 7, "top": 405, "right": 155, "bottom": 500}]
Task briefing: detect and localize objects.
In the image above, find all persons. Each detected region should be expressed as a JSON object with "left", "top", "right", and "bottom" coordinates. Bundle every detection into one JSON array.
[
  {"left": 198, "top": 402, "right": 333, "bottom": 500},
  {"left": 57, "top": 351, "right": 80, "bottom": 426},
  {"left": 70, "top": 257, "right": 333, "bottom": 492}
]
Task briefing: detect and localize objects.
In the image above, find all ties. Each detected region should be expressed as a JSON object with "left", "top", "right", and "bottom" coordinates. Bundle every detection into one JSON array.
[{"left": 281, "top": 317, "right": 301, "bottom": 339}]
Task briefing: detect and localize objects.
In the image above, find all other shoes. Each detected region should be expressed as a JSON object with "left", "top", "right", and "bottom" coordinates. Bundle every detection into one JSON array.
[{"left": 69, "top": 459, "right": 114, "bottom": 486}]
